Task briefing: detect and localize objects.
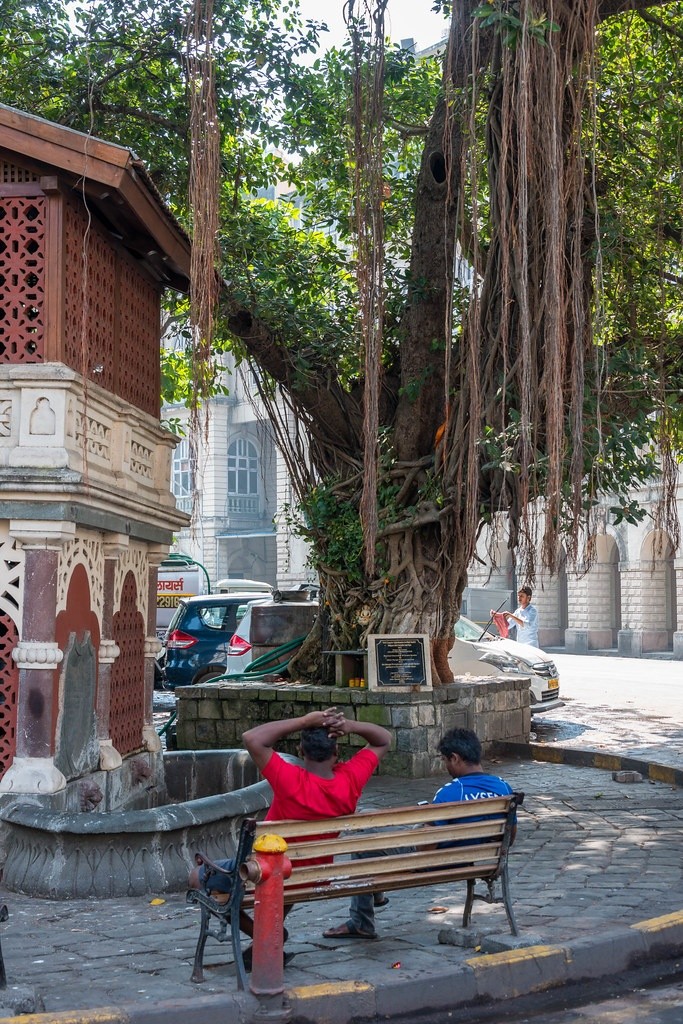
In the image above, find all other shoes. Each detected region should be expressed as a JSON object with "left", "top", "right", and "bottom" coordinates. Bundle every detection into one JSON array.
[{"left": 242, "top": 926, "right": 296, "bottom": 974}]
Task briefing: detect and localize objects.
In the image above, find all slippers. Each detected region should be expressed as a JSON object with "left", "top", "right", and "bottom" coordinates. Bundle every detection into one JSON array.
[
  {"left": 322, "top": 919, "right": 378, "bottom": 939},
  {"left": 373, "top": 897, "right": 389, "bottom": 907}
]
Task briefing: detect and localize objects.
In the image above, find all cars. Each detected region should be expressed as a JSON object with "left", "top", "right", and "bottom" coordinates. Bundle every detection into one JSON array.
[
  {"left": 162, "top": 591, "right": 274, "bottom": 691},
  {"left": 449, "top": 614, "right": 566, "bottom": 714}
]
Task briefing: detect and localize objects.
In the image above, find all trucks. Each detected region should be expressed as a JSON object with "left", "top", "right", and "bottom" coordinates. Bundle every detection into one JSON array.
[{"left": 152, "top": 554, "right": 273, "bottom": 683}]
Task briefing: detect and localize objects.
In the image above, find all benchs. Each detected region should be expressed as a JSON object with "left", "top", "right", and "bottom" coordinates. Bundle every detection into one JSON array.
[{"left": 187, "top": 790, "right": 528, "bottom": 992}]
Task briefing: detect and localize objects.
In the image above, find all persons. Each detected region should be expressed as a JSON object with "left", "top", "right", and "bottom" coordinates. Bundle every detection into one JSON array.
[
  {"left": 322, "top": 726, "right": 518, "bottom": 939},
  {"left": 490, "top": 586, "right": 539, "bottom": 648},
  {"left": 188, "top": 706, "right": 392, "bottom": 973}
]
